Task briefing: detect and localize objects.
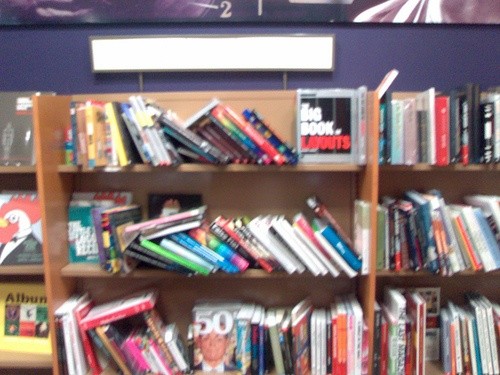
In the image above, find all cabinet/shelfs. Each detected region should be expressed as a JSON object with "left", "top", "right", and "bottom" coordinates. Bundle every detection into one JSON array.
[{"left": 0, "top": 86, "right": 500, "bottom": 375}]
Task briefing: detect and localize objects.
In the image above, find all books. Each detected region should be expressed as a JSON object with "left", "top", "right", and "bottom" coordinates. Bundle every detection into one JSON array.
[
  {"left": 55, "top": 287, "right": 368, "bottom": 375},
  {"left": 0, "top": 283, "right": 53, "bottom": 368},
  {"left": 65, "top": 95, "right": 298, "bottom": 167},
  {"left": 0, "top": 91, "right": 55, "bottom": 166},
  {"left": 376, "top": 69, "right": 500, "bottom": 167},
  {"left": 67, "top": 192, "right": 371, "bottom": 280},
  {"left": 296, "top": 88, "right": 366, "bottom": 166},
  {"left": 0, "top": 189, "right": 43, "bottom": 264},
  {"left": 376, "top": 189, "right": 500, "bottom": 276},
  {"left": 372, "top": 286, "right": 500, "bottom": 375}
]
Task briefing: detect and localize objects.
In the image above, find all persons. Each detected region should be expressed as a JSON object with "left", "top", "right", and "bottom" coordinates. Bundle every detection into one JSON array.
[{"left": 194, "top": 324, "right": 233, "bottom": 371}]
